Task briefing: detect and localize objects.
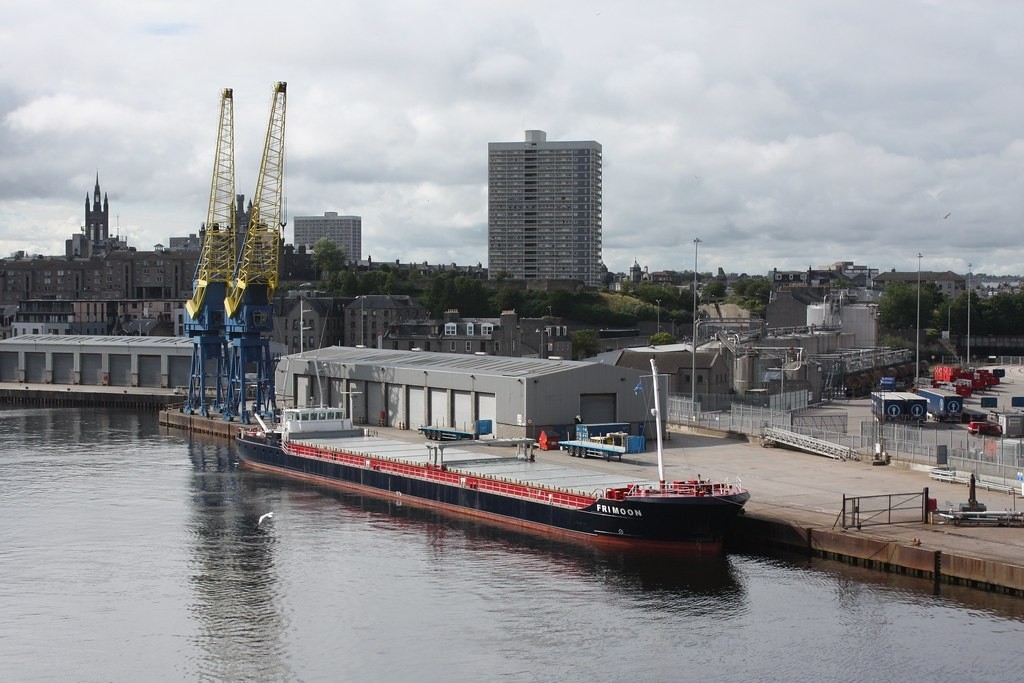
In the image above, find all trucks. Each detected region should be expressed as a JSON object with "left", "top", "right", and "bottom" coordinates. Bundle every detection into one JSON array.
[
  {"left": 878, "top": 377, "right": 896, "bottom": 391},
  {"left": 968, "top": 422, "right": 1002, "bottom": 437}
]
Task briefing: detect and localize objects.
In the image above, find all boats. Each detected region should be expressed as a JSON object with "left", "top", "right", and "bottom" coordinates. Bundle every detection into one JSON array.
[{"left": 234, "top": 360, "right": 751, "bottom": 560}]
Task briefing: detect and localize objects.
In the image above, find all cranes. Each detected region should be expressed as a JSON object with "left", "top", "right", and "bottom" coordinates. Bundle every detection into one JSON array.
[{"left": 183, "top": 77, "right": 288, "bottom": 426}]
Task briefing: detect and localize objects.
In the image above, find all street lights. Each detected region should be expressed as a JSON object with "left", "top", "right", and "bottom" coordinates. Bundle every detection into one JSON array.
[
  {"left": 356, "top": 295, "right": 367, "bottom": 345},
  {"left": 916, "top": 252, "right": 923, "bottom": 383},
  {"left": 692, "top": 237, "right": 702, "bottom": 413},
  {"left": 967, "top": 262, "right": 973, "bottom": 364},
  {"left": 656, "top": 299, "right": 661, "bottom": 334}
]
